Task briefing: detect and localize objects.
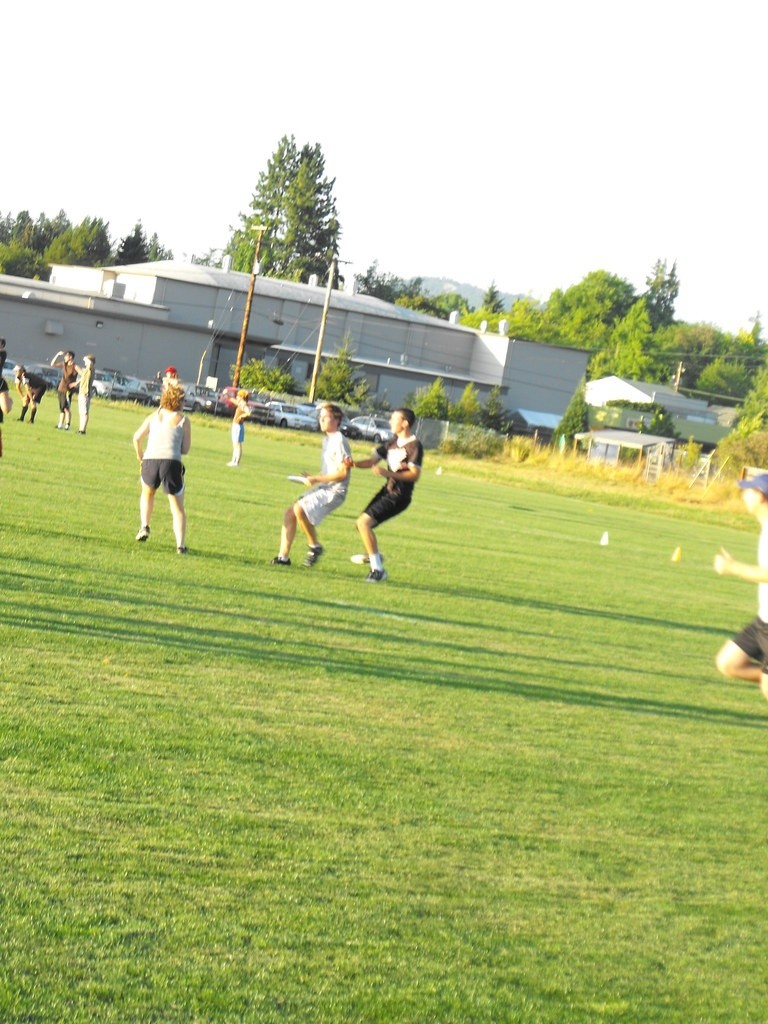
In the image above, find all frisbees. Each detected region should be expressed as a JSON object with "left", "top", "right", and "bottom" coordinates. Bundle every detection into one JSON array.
[{"left": 287, "top": 475, "right": 309, "bottom": 483}]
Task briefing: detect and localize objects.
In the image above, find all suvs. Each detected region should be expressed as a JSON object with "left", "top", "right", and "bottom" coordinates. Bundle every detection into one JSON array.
[
  {"left": 222, "top": 388, "right": 273, "bottom": 423},
  {"left": 181, "top": 382, "right": 227, "bottom": 416}
]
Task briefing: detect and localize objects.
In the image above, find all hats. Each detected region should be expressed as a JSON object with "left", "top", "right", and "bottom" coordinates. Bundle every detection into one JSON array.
[
  {"left": 238, "top": 390, "right": 250, "bottom": 397},
  {"left": 164, "top": 367, "right": 176, "bottom": 374},
  {"left": 737, "top": 473, "right": 768, "bottom": 496}
]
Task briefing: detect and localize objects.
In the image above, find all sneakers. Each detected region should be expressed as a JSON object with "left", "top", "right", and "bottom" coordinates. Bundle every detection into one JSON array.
[
  {"left": 136, "top": 525, "right": 150, "bottom": 542},
  {"left": 365, "top": 568, "right": 386, "bottom": 582},
  {"left": 270, "top": 556, "right": 291, "bottom": 566},
  {"left": 177, "top": 546, "right": 188, "bottom": 554},
  {"left": 302, "top": 544, "right": 323, "bottom": 567},
  {"left": 350, "top": 554, "right": 382, "bottom": 564}
]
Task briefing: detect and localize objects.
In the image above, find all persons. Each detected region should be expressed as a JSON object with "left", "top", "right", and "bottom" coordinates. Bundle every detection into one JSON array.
[
  {"left": 0, "top": 338, "right": 13, "bottom": 456},
  {"left": 75, "top": 354, "right": 96, "bottom": 436},
  {"left": 269, "top": 404, "right": 351, "bottom": 567},
  {"left": 341, "top": 408, "right": 424, "bottom": 583},
  {"left": 132, "top": 384, "right": 192, "bottom": 555},
  {"left": 50, "top": 350, "right": 83, "bottom": 431},
  {"left": 13, "top": 364, "right": 47, "bottom": 424},
  {"left": 712, "top": 473, "right": 768, "bottom": 702},
  {"left": 223, "top": 389, "right": 251, "bottom": 467},
  {"left": 157, "top": 367, "right": 179, "bottom": 389}
]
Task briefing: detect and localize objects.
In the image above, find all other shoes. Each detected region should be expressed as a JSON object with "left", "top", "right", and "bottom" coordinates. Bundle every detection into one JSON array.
[
  {"left": 64, "top": 423, "right": 70, "bottom": 430},
  {"left": 226, "top": 461, "right": 239, "bottom": 467},
  {"left": 17, "top": 418, "right": 23, "bottom": 421},
  {"left": 55, "top": 425, "right": 63, "bottom": 428},
  {"left": 27, "top": 419, "right": 33, "bottom": 423},
  {"left": 76, "top": 431, "right": 85, "bottom": 434}
]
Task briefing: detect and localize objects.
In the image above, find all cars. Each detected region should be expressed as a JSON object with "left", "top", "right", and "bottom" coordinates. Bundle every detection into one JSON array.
[
  {"left": 77, "top": 369, "right": 124, "bottom": 399},
  {"left": 110, "top": 370, "right": 134, "bottom": 383},
  {"left": 352, "top": 416, "right": 396, "bottom": 442},
  {"left": 267, "top": 401, "right": 318, "bottom": 431},
  {"left": 308, "top": 406, "right": 357, "bottom": 439},
  {"left": 2, "top": 360, "right": 21, "bottom": 381},
  {"left": 126, "top": 379, "right": 162, "bottom": 405},
  {"left": 25, "top": 365, "right": 61, "bottom": 387}
]
